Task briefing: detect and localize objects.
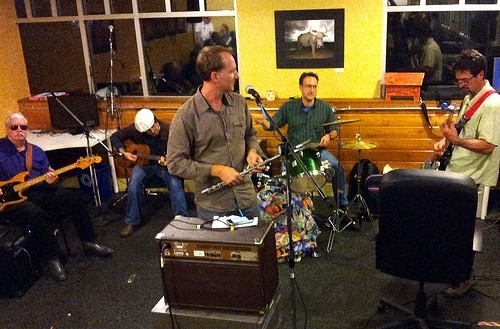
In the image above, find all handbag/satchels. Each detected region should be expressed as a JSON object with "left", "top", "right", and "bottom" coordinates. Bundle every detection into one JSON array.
[{"left": 0, "top": 223, "right": 38, "bottom": 296}]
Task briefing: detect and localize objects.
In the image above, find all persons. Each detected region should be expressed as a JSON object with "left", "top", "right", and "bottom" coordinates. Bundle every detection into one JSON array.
[
  {"left": 167, "top": 45, "right": 268, "bottom": 223},
  {"left": 0, "top": 113, "right": 114, "bottom": 282},
  {"left": 182, "top": 50, "right": 204, "bottom": 86},
  {"left": 255, "top": 71, "right": 349, "bottom": 212},
  {"left": 110, "top": 108, "right": 188, "bottom": 237},
  {"left": 433, "top": 51, "right": 500, "bottom": 295},
  {"left": 412, "top": 24, "right": 443, "bottom": 83},
  {"left": 158, "top": 62, "right": 196, "bottom": 95},
  {"left": 217, "top": 24, "right": 231, "bottom": 44},
  {"left": 193, "top": 16, "right": 215, "bottom": 45}
]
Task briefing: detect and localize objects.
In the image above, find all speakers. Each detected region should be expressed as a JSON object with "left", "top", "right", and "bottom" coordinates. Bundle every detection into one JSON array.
[
  {"left": 157, "top": 215, "right": 279, "bottom": 313},
  {"left": 375, "top": 169, "right": 479, "bottom": 284}
]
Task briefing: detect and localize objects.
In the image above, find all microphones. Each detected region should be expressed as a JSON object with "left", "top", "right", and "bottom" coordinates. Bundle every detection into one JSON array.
[
  {"left": 246, "top": 85, "right": 262, "bottom": 101},
  {"left": 108, "top": 24, "right": 114, "bottom": 32}
]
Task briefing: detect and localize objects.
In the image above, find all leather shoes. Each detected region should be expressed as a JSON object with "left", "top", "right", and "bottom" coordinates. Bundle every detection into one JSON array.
[
  {"left": 83, "top": 241, "right": 114, "bottom": 255},
  {"left": 48, "top": 256, "right": 68, "bottom": 281}
]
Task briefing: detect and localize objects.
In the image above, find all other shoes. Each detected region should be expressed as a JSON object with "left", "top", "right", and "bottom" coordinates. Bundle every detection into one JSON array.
[
  {"left": 340, "top": 206, "right": 356, "bottom": 221},
  {"left": 119, "top": 224, "right": 134, "bottom": 237},
  {"left": 444, "top": 277, "right": 477, "bottom": 297}
]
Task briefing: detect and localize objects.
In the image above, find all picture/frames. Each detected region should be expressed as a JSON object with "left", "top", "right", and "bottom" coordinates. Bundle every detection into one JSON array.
[{"left": 274, "top": 8, "right": 345, "bottom": 69}]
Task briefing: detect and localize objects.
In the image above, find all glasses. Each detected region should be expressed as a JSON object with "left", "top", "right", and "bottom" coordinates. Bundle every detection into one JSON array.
[
  {"left": 6, "top": 125, "right": 28, "bottom": 130},
  {"left": 453, "top": 75, "right": 474, "bottom": 84}
]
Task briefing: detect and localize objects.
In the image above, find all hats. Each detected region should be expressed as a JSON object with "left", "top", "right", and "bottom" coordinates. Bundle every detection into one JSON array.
[{"left": 134, "top": 108, "right": 154, "bottom": 132}]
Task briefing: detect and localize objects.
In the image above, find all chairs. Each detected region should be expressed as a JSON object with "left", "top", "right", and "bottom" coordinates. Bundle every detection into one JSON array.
[
  {"left": 143, "top": 176, "right": 185, "bottom": 205},
  {"left": 375, "top": 168, "right": 483, "bottom": 329}
]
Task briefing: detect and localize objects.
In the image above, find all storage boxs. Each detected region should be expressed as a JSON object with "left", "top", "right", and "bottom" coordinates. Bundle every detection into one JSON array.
[{"left": 17, "top": 91, "right": 99, "bottom": 131}]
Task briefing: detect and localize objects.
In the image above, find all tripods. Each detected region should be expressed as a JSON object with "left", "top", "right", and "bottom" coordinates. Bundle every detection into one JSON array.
[{"left": 326, "top": 124, "right": 375, "bottom": 252}]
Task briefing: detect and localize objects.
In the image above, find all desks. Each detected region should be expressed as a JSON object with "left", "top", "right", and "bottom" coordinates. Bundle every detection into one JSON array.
[{"left": 24, "top": 129, "right": 119, "bottom": 208}]
